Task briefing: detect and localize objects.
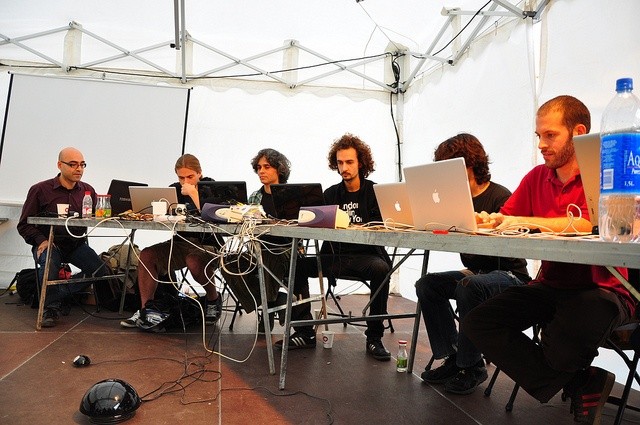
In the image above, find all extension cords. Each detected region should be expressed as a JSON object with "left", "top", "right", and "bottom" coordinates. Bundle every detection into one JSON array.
[{"left": 153, "top": 214, "right": 187, "bottom": 222}]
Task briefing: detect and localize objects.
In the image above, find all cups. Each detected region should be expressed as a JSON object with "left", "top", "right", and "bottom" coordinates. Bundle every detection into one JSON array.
[
  {"left": 322, "top": 330, "right": 334, "bottom": 348},
  {"left": 57, "top": 203, "right": 69, "bottom": 218},
  {"left": 151, "top": 201, "right": 167, "bottom": 216}
]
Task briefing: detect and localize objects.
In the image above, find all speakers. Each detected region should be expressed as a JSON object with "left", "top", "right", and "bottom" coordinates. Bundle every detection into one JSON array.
[
  {"left": 201, "top": 202, "right": 230, "bottom": 224},
  {"left": 298, "top": 204, "right": 350, "bottom": 229}
]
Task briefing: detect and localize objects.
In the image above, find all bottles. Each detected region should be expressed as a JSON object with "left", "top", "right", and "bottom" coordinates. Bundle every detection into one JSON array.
[
  {"left": 82, "top": 191, "right": 93, "bottom": 218},
  {"left": 100, "top": 196, "right": 105, "bottom": 216},
  {"left": 598, "top": 79, "right": 636, "bottom": 243},
  {"left": 104, "top": 194, "right": 111, "bottom": 217},
  {"left": 397, "top": 341, "right": 409, "bottom": 372},
  {"left": 95, "top": 195, "right": 102, "bottom": 216}
]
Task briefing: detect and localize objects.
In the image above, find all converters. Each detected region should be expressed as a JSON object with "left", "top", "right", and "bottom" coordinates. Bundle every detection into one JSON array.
[{"left": 152, "top": 202, "right": 167, "bottom": 215}]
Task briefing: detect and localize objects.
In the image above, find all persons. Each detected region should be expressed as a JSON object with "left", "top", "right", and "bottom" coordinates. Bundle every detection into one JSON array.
[
  {"left": 120, "top": 154, "right": 226, "bottom": 328},
  {"left": 16, "top": 147, "right": 106, "bottom": 327},
  {"left": 415, "top": 133, "right": 532, "bottom": 394},
  {"left": 461, "top": 94, "right": 636, "bottom": 424},
  {"left": 275, "top": 132, "right": 393, "bottom": 360},
  {"left": 217, "top": 148, "right": 307, "bottom": 333}
]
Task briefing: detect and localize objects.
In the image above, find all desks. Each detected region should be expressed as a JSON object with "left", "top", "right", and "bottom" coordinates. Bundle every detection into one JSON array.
[
  {"left": 26, "top": 215, "right": 331, "bottom": 377},
  {"left": 269, "top": 223, "right": 639, "bottom": 423}
]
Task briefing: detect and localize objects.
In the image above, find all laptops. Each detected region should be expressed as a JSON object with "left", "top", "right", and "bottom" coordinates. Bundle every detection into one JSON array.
[
  {"left": 128, "top": 185, "right": 177, "bottom": 215},
  {"left": 269, "top": 183, "right": 326, "bottom": 220},
  {"left": 107, "top": 180, "right": 148, "bottom": 216},
  {"left": 197, "top": 180, "right": 248, "bottom": 212},
  {"left": 403, "top": 157, "right": 499, "bottom": 235},
  {"left": 572, "top": 128, "right": 639, "bottom": 227},
  {"left": 372, "top": 182, "right": 412, "bottom": 227}
]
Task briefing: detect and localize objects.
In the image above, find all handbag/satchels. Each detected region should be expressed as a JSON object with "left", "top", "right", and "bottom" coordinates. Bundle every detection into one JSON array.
[
  {"left": 140, "top": 297, "right": 201, "bottom": 334},
  {"left": 14, "top": 269, "right": 44, "bottom": 305},
  {"left": 100, "top": 244, "right": 141, "bottom": 287}
]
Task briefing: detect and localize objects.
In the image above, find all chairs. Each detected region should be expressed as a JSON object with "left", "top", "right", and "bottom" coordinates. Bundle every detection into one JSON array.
[
  {"left": 504, "top": 267, "right": 639, "bottom": 423},
  {"left": 31, "top": 232, "right": 102, "bottom": 314},
  {"left": 227, "top": 239, "right": 312, "bottom": 332},
  {"left": 178, "top": 267, "right": 244, "bottom": 317},
  {"left": 424, "top": 297, "right": 525, "bottom": 396},
  {"left": 319, "top": 276, "right": 395, "bottom": 334}
]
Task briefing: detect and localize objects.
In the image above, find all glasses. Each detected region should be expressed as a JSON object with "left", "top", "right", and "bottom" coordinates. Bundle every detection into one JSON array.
[{"left": 59, "top": 160, "right": 86, "bottom": 168}]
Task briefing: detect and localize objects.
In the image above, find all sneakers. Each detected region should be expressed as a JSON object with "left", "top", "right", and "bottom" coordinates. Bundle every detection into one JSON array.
[
  {"left": 443, "top": 359, "right": 488, "bottom": 394},
  {"left": 367, "top": 339, "right": 391, "bottom": 360},
  {"left": 421, "top": 355, "right": 460, "bottom": 383},
  {"left": 41, "top": 308, "right": 59, "bottom": 326},
  {"left": 274, "top": 332, "right": 316, "bottom": 349},
  {"left": 120, "top": 310, "right": 140, "bottom": 328},
  {"left": 276, "top": 292, "right": 287, "bottom": 325},
  {"left": 259, "top": 306, "right": 274, "bottom": 333},
  {"left": 205, "top": 292, "right": 222, "bottom": 324},
  {"left": 563, "top": 366, "right": 615, "bottom": 425}
]
